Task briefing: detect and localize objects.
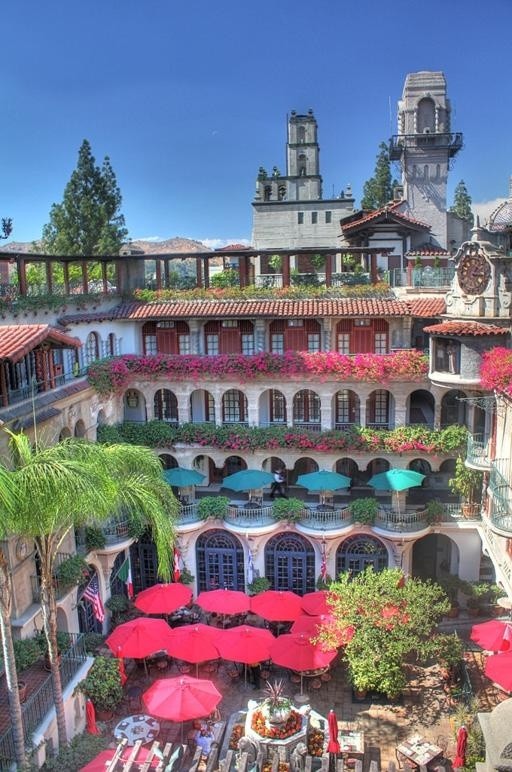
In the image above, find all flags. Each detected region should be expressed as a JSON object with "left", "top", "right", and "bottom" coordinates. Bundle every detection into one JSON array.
[
  {"left": 83, "top": 571, "right": 105, "bottom": 624},
  {"left": 117, "top": 558, "right": 134, "bottom": 600},
  {"left": 247, "top": 547, "right": 255, "bottom": 586},
  {"left": 172, "top": 545, "right": 184, "bottom": 584},
  {"left": 320, "top": 548, "right": 328, "bottom": 583}
]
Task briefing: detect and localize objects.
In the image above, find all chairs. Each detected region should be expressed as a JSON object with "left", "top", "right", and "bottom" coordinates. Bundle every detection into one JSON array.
[{"left": 395, "top": 731, "right": 447, "bottom": 765}]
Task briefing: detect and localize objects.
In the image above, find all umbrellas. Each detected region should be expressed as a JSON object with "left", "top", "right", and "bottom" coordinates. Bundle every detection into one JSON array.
[
  {"left": 469, "top": 618, "right": 512, "bottom": 653},
  {"left": 80, "top": 744, "right": 162, "bottom": 771},
  {"left": 106, "top": 581, "right": 399, "bottom": 697},
  {"left": 163, "top": 467, "right": 207, "bottom": 498},
  {"left": 326, "top": 709, "right": 341, "bottom": 772},
  {"left": 451, "top": 725, "right": 468, "bottom": 772},
  {"left": 483, "top": 652, "right": 512, "bottom": 694},
  {"left": 142, "top": 673, "right": 222, "bottom": 767},
  {"left": 222, "top": 468, "right": 275, "bottom": 502},
  {"left": 366, "top": 467, "right": 427, "bottom": 522},
  {"left": 296, "top": 469, "right": 352, "bottom": 504},
  {"left": 86, "top": 698, "right": 97, "bottom": 735}
]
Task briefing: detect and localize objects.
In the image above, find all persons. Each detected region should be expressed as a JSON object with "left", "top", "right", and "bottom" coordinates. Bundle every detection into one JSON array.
[
  {"left": 269, "top": 468, "right": 288, "bottom": 501},
  {"left": 193, "top": 728, "right": 214, "bottom": 760},
  {"left": 207, "top": 708, "right": 221, "bottom": 723}
]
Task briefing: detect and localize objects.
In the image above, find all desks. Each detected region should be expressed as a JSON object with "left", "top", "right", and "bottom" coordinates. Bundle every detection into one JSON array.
[
  {"left": 332, "top": 729, "right": 364, "bottom": 755},
  {"left": 112, "top": 712, "right": 160, "bottom": 745},
  {"left": 396, "top": 732, "right": 443, "bottom": 772},
  {"left": 291, "top": 663, "right": 330, "bottom": 697},
  {"left": 191, "top": 718, "right": 227, "bottom": 747}
]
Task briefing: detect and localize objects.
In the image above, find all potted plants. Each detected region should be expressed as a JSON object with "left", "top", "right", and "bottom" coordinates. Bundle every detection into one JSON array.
[
  {"left": 0, "top": 627, "right": 123, "bottom": 720},
  {"left": 448, "top": 451, "right": 482, "bottom": 519},
  {"left": 440, "top": 573, "right": 505, "bottom": 619},
  {"left": 346, "top": 658, "right": 406, "bottom": 704}
]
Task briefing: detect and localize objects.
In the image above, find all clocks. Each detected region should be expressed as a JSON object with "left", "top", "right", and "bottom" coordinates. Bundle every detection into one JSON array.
[{"left": 457, "top": 252, "right": 489, "bottom": 295}]
